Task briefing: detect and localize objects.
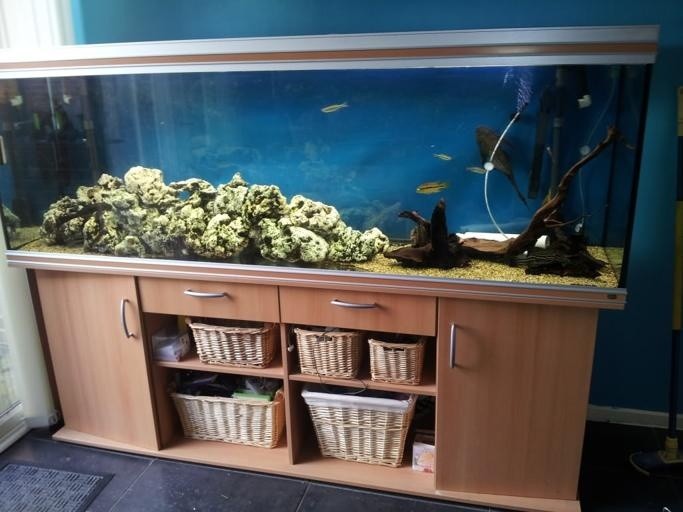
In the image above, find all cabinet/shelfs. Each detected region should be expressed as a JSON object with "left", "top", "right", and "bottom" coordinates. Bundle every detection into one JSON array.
[
  {"left": 435, "top": 296, "right": 599, "bottom": 501},
  {"left": 138, "top": 276, "right": 291, "bottom": 480},
  {"left": 279, "top": 285, "right": 436, "bottom": 500},
  {"left": 34, "top": 269, "right": 162, "bottom": 451}
]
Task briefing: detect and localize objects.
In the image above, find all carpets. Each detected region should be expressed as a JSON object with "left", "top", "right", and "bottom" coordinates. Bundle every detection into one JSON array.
[{"left": 1, "top": 461, "right": 117, "bottom": 512}]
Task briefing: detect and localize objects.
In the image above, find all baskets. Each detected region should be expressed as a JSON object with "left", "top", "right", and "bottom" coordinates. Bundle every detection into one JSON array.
[
  {"left": 169, "top": 386, "right": 285, "bottom": 450},
  {"left": 366, "top": 332, "right": 428, "bottom": 385},
  {"left": 293, "top": 327, "right": 367, "bottom": 381},
  {"left": 301, "top": 383, "right": 419, "bottom": 468},
  {"left": 184, "top": 316, "right": 280, "bottom": 369}
]
